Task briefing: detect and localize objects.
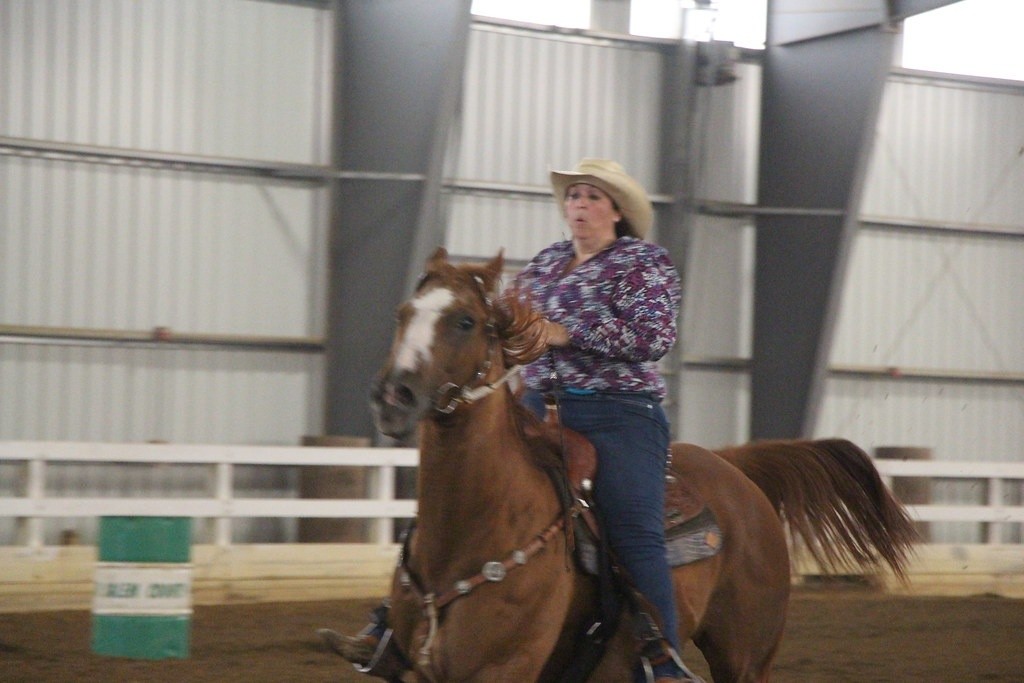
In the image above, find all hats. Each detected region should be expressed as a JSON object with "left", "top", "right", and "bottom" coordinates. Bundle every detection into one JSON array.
[{"left": 551, "top": 155, "right": 652, "bottom": 241}]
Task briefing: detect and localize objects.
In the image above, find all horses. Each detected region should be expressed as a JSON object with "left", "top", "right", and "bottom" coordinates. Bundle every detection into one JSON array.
[{"left": 373, "top": 246, "right": 928, "bottom": 683}]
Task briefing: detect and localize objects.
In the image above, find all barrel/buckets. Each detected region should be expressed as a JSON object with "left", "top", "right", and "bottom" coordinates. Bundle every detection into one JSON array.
[{"left": 89, "top": 515, "right": 193, "bottom": 660}]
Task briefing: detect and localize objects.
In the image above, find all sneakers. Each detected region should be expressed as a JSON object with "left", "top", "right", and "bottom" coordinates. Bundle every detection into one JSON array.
[{"left": 320, "top": 627, "right": 402, "bottom": 681}]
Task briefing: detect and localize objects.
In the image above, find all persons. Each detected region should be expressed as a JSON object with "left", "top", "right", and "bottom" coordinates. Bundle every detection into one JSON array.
[{"left": 315, "top": 158, "right": 690, "bottom": 683}]
